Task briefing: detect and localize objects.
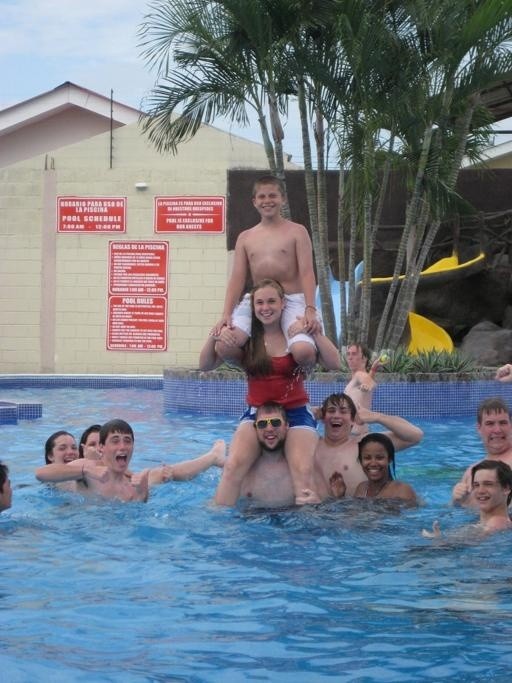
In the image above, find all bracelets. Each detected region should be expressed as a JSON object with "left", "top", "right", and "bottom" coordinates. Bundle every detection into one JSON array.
[
  {"left": 370, "top": 367, "right": 378, "bottom": 372},
  {"left": 305, "top": 306, "right": 317, "bottom": 311}
]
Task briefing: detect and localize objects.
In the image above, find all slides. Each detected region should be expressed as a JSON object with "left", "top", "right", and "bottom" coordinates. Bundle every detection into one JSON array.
[
  {"left": 354, "top": 253, "right": 486, "bottom": 355},
  {"left": 315, "top": 258, "right": 365, "bottom": 347}
]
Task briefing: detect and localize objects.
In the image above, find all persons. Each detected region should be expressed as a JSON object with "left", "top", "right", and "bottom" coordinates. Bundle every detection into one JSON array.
[
  {"left": 420, "top": 460, "right": 512, "bottom": 544},
  {"left": 343, "top": 342, "right": 392, "bottom": 440},
  {"left": 207, "top": 174, "right": 324, "bottom": 368},
  {"left": 0, "top": 462, "right": 13, "bottom": 515},
  {"left": 32, "top": 418, "right": 227, "bottom": 505},
  {"left": 450, "top": 364, "right": 512, "bottom": 511},
  {"left": 238, "top": 393, "right": 422, "bottom": 509},
  {"left": 198, "top": 276, "right": 342, "bottom": 510}
]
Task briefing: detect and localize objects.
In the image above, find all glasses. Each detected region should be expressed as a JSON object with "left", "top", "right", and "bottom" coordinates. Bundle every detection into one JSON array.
[{"left": 255, "top": 418, "right": 282, "bottom": 429}]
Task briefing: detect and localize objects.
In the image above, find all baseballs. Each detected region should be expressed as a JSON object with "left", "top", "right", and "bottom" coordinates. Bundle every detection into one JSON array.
[{"left": 380, "top": 355, "right": 388, "bottom": 362}]
